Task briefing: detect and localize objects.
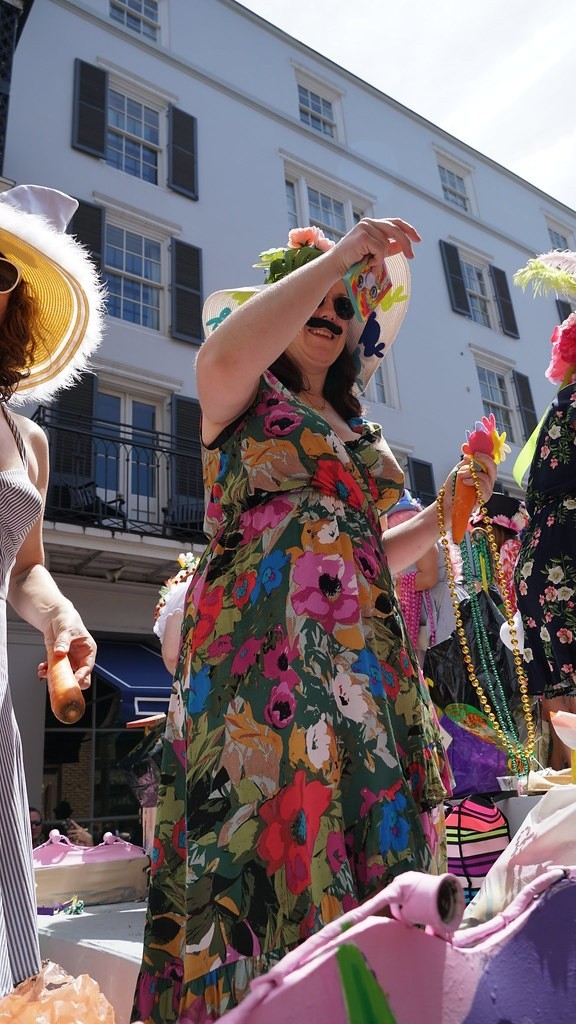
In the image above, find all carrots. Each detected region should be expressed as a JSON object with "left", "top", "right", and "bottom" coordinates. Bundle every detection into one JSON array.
[
  {"left": 45, "top": 649, "right": 86, "bottom": 725},
  {"left": 452, "top": 463, "right": 477, "bottom": 544}
]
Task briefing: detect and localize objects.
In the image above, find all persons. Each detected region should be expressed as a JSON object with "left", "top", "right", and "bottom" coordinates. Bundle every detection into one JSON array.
[
  {"left": 130, "top": 221, "right": 495, "bottom": 1024},
  {"left": 514, "top": 310, "right": 575, "bottom": 772},
  {"left": 30, "top": 807, "right": 93, "bottom": 849},
  {"left": 0, "top": 185, "right": 106, "bottom": 1002},
  {"left": 378, "top": 489, "right": 438, "bottom": 596}
]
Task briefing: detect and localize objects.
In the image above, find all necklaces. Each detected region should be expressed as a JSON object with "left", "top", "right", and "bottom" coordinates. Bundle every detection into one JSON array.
[
  {"left": 436, "top": 456, "right": 536, "bottom": 779},
  {"left": 399, "top": 571, "right": 435, "bottom": 652}
]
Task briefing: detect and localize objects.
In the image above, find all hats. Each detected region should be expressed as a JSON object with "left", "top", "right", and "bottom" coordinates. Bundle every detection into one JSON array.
[
  {"left": 470, "top": 493, "right": 522, "bottom": 537},
  {"left": 0, "top": 185, "right": 108, "bottom": 401},
  {"left": 388, "top": 489, "right": 423, "bottom": 518},
  {"left": 201, "top": 237, "right": 413, "bottom": 397}
]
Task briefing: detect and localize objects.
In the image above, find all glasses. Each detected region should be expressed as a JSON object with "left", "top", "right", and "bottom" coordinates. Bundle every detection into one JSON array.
[
  {"left": 316, "top": 296, "right": 355, "bottom": 320},
  {"left": 0, "top": 257, "right": 24, "bottom": 295}
]
